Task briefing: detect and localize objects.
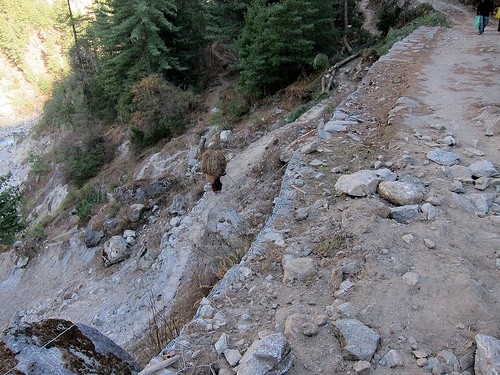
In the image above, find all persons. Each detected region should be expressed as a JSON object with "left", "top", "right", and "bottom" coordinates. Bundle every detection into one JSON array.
[
  {"left": 495, "top": 4, "right": 500, "bottom": 34},
  {"left": 476, "top": 0, "right": 494, "bottom": 35}
]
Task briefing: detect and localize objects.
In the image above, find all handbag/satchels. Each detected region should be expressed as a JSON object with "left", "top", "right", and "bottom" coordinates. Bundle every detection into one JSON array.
[{"left": 475, "top": 15, "right": 480, "bottom": 29}]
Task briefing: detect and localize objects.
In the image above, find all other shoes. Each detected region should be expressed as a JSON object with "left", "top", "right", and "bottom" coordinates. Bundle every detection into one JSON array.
[
  {"left": 497, "top": 31, "right": 500, "bottom": 35},
  {"left": 478, "top": 32, "right": 482, "bottom": 35}
]
